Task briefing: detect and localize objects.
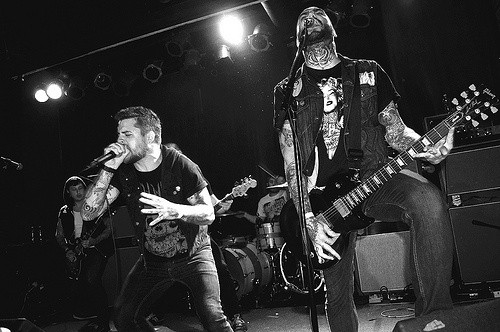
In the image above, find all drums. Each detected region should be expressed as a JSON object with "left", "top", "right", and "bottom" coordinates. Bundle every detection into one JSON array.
[
  {"left": 214, "top": 242, "right": 276, "bottom": 299},
  {"left": 221, "top": 235, "right": 251, "bottom": 247},
  {"left": 255, "top": 221, "right": 285, "bottom": 250},
  {"left": 272, "top": 243, "right": 323, "bottom": 295}
]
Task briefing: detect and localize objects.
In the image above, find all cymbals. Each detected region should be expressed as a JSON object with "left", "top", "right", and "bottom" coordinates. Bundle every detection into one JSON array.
[{"left": 266, "top": 182, "right": 289, "bottom": 188}]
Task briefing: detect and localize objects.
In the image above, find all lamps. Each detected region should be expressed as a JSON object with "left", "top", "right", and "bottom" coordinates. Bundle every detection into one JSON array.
[{"left": 33, "top": 0, "right": 370, "bottom": 103}]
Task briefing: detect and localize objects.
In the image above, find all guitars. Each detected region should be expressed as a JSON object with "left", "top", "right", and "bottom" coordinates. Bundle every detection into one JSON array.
[
  {"left": 62, "top": 204, "right": 117, "bottom": 280},
  {"left": 214, "top": 174, "right": 257, "bottom": 212},
  {"left": 279, "top": 83, "right": 500, "bottom": 269}
]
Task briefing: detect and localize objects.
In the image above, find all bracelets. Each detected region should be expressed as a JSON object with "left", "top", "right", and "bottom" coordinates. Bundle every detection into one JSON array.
[{"left": 101, "top": 166, "right": 117, "bottom": 175}]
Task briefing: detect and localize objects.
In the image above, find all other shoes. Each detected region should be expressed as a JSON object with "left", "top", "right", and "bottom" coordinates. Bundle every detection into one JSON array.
[{"left": 232, "top": 314, "right": 248, "bottom": 332}]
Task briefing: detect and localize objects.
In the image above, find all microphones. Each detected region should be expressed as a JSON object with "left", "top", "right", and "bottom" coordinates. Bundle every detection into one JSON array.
[
  {"left": 87, "top": 145, "right": 125, "bottom": 169},
  {"left": 0, "top": 156, "right": 23, "bottom": 171}
]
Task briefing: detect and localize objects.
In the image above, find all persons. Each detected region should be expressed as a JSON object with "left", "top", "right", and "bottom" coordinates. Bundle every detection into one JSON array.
[
  {"left": 132, "top": 139, "right": 249, "bottom": 332},
  {"left": 270, "top": 6, "right": 459, "bottom": 332},
  {"left": 54, "top": 175, "right": 116, "bottom": 321},
  {"left": 78, "top": 104, "right": 237, "bottom": 332},
  {"left": 237, "top": 171, "right": 292, "bottom": 226}
]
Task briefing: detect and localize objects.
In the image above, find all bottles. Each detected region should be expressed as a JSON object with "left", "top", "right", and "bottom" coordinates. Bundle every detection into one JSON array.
[
  {"left": 439, "top": 93, "right": 452, "bottom": 112},
  {"left": 29, "top": 225, "right": 44, "bottom": 241}
]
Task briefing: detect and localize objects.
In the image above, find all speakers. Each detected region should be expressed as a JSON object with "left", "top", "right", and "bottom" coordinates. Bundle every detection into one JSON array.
[
  {"left": 0, "top": 318, "right": 45, "bottom": 332},
  {"left": 352, "top": 230, "right": 415, "bottom": 293},
  {"left": 431, "top": 140, "right": 500, "bottom": 286},
  {"left": 108, "top": 205, "right": 153, "bottom": 295}
]
